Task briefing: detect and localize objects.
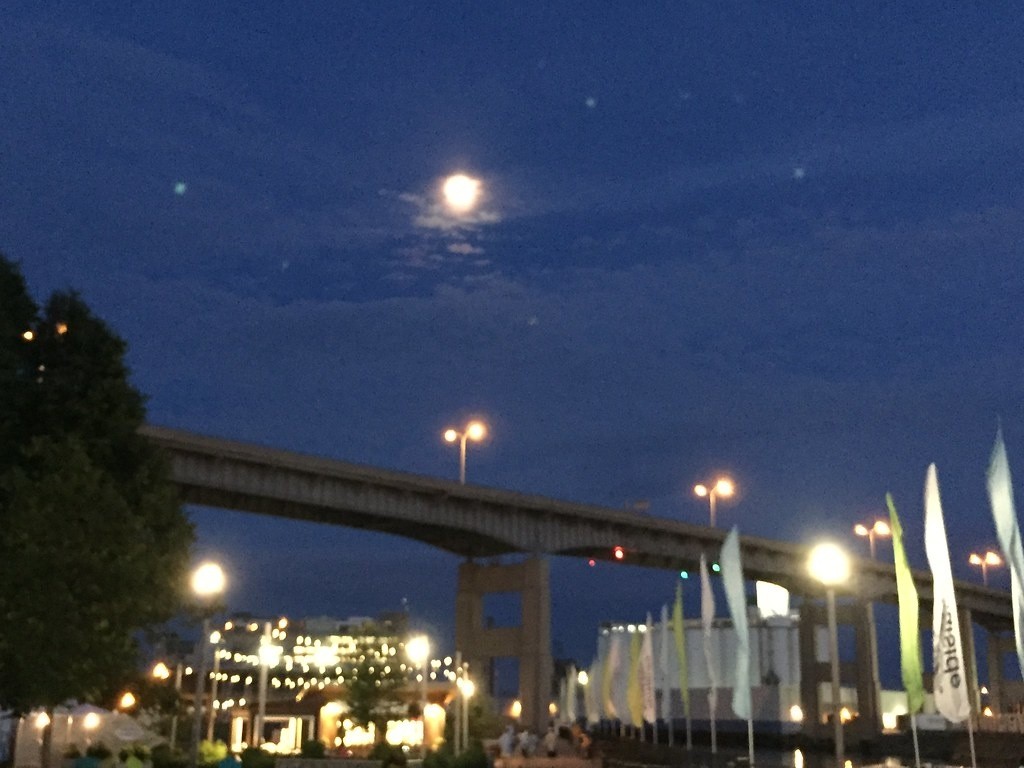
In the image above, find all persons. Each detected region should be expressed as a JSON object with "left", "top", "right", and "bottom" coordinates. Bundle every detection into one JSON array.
[
  {"left": 571, "top": 721, "right": 592, "bottom": 760},
  {"left": 498, "top": 725, "right": 557, "bottom": 768}
]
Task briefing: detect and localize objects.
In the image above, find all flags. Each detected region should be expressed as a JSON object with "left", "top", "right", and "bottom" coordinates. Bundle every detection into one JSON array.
[
  {"left": 885, "top": 492, "right": 927, "bottom": 717},
  {"left": 985, "top": 424, "right": 1024, "bottom": 680},
  {"left": 673, "top": 584, "right": 689, "bottom": 716},
  {"left": 586, "top": 606, "right": 672, "bottom": 728},
  {"left": 924, "top": 464, "right": 971, "bottom": 724},
  {"left": 699, "top": 553, "right": 715, "bottom": 713},
  {"left": 720, "top": 525, "right": 752, "bottom": 719},
  {"left": 560, "top": 664, "right": 578, "bottom": 724}
]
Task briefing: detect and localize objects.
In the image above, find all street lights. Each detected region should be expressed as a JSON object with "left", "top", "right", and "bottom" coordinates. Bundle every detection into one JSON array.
[
  {"left": 188, "top": 560, "right": 226, "bottom": 767},
  {"left": 696, "top": 477, "right": 733, "bottom": 531},
  {"left": 969, "top": 551, "right": 1002, "bottom": 589},
  {"left": 444, "top": 422, "right": 486, "bottom": 485},
  {"left": 854, "top": 521, "right": 890, "bottom": 560},
  {"left": 806, "top": 538, "right": 854, "bottom": 768}
]
